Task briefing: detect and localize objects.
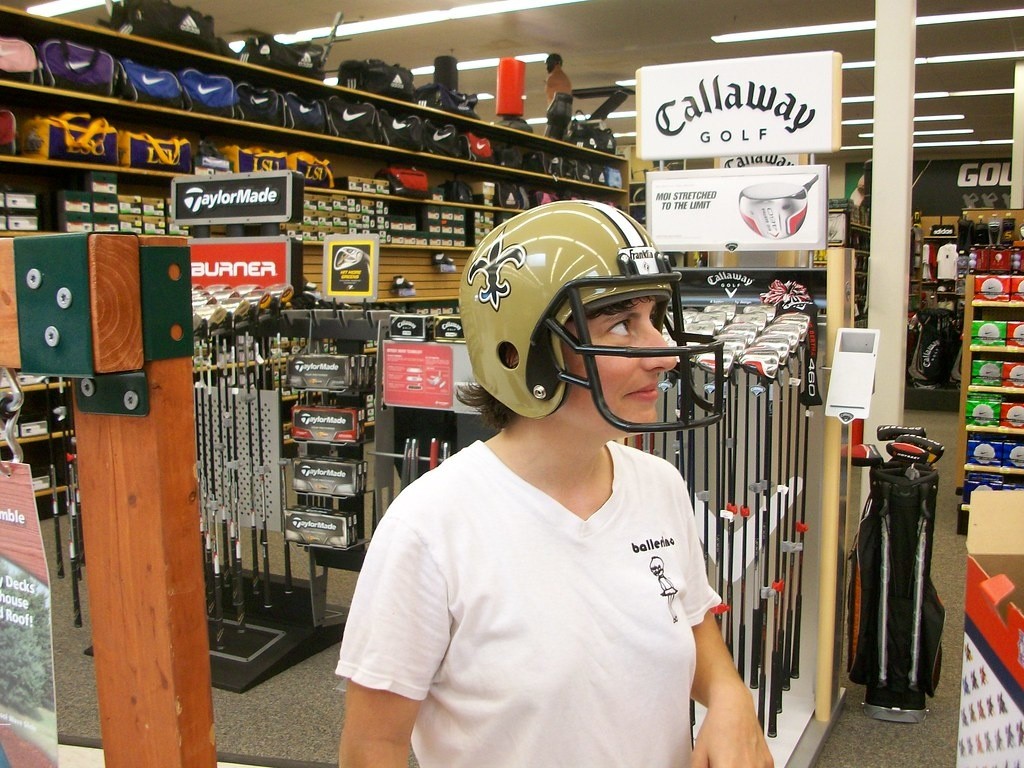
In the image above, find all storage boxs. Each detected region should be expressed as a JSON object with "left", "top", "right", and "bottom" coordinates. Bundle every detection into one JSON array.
[
  {"left": 390, "top": 313, "right": 434, "bottom": 341},
  {"left": 434, "top": 314, "right": 465, "bottom": 343},
  {"left": 0, "top": 176, "right": 494, "bottom": 246}
]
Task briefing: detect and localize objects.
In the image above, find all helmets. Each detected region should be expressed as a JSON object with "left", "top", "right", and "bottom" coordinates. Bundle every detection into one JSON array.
[{"left": 459, "top": 200, "right": 674, "bottom": 420}]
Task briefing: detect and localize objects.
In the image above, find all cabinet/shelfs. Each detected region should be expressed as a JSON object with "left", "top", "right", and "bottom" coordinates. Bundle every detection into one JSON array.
[
  {"left": 955, "top": 276, "right": 1024, "bottom": 533},
  {"left": 0, "top": 4, "right": 629, "bottom": 304}
]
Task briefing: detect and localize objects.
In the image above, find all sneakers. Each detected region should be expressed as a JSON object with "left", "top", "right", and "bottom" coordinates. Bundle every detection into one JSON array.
[
  {"left": 391, "top": 276, "right": 413, "bottom": 290},
  {"left": 431, "top": 253, "right": 454, "bottom": 265}
]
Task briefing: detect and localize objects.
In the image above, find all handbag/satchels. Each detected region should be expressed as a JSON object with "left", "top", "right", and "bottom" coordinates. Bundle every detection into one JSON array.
[{"left": 0, "top": 0, "right": 648, "bottom": 226}]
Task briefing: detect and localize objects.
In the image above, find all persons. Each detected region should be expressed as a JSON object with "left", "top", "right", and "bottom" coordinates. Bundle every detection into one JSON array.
[{"left": 338, "top": 198, "right": 775, "bottom": 768}]
